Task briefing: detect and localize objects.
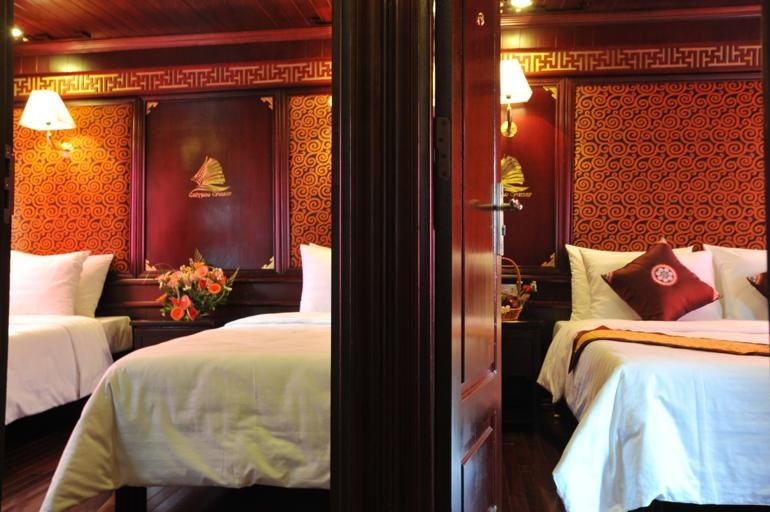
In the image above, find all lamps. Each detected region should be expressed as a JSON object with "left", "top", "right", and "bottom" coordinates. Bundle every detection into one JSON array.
[
  {"left": 18, "top": 89, "right": 78, "bottom": 154},
  {"left": 501, "top": 61, "right": 533, "bottom": 138}
]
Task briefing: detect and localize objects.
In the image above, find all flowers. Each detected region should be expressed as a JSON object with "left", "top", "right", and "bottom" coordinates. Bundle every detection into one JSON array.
[
  {"left": 496, "top": 279, "right": 537, "bottom": 307},
  {"left": 141, "top": 246, "right": 242, "bottom": 322}
]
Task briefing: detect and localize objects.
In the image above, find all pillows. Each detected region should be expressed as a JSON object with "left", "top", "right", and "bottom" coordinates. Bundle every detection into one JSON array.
[
  {"left": 297, "top": 241, "right": 332, "bottom": 312},
  {"left": 8, "top": 249, "right": 115, "bottom": 319},
  {"left": 565, "top": 244, "right": 769, "bottom": 321}
]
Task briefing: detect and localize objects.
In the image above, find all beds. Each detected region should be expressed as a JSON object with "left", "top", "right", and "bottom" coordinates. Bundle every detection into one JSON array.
[{"left": 539, "top": 319, "right": 770, "bottom": 512}]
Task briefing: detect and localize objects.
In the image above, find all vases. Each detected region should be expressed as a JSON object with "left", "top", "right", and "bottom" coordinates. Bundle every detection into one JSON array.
[{"left": 502, "top": 309, "right": 522, "bottom": 321}]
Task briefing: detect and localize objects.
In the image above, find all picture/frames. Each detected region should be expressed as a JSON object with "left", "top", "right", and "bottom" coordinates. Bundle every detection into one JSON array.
[
  {"left": 502, "top": 79, "right": 574, "bottom": 284},
  {"left": 129, "top": 96, "right": 290, "bottom": 275}
]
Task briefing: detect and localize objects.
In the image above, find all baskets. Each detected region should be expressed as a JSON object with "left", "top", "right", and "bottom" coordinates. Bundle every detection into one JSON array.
[{"left": 502, "top": 256, "right": 523, "bottom": 320}]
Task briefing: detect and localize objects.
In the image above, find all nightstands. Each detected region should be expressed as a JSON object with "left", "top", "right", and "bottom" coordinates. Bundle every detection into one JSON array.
[{"left": 503, "top": 319, "right": 550, "bottom": 429}]
